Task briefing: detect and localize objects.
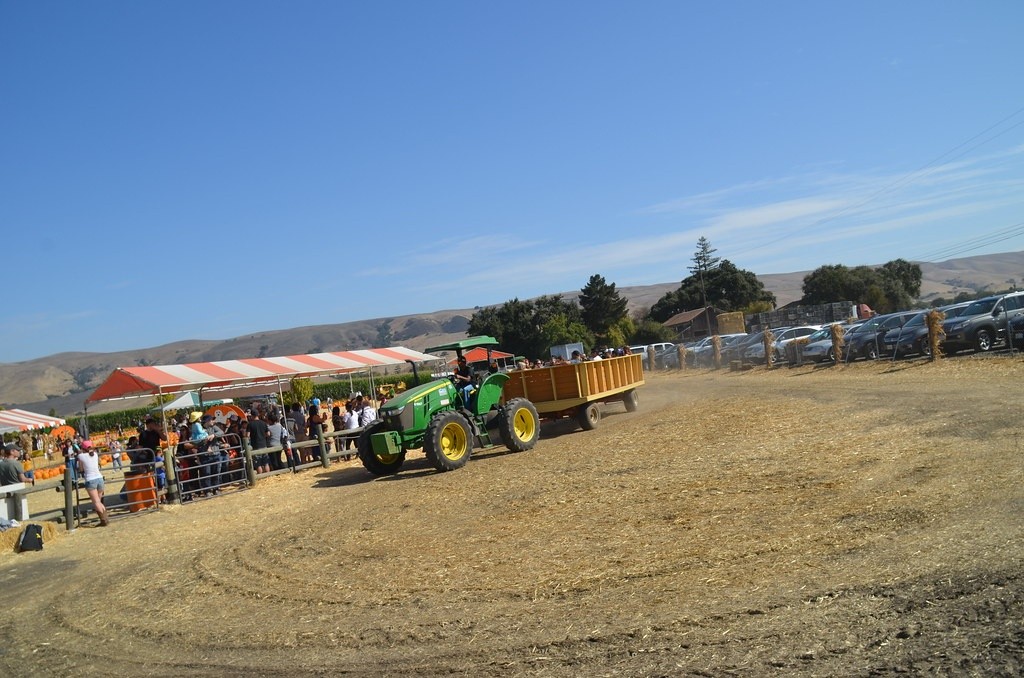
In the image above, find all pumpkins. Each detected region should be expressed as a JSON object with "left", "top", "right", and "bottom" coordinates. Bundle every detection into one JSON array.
[
  {"left": 33, "top": 452, "right": 128, "bottom": 479},
  {"left": 159, "top": 431, "right": 179, "bottom": 448},
  {"left": 90, "top": 431, "right": 138, "bottom": 446}
]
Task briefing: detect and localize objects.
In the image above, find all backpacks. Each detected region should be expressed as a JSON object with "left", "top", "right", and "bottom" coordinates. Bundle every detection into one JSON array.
[{"left": 18, "top": 524, "right": 45, "bottom": 552}]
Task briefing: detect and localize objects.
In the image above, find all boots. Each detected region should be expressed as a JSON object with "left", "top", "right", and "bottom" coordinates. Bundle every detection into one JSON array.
[{"left": 95, "top": 507, "right": 110, "bottom": 527}]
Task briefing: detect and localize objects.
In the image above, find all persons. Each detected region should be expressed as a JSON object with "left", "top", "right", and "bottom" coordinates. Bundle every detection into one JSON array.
[
  {"left": 510, "top": 345, "right": 632, "bottom": 371},
  {"left": 77, "top": 441, "right": 110, "bottom": 527},
  {"left": 453, "top": 356, "right": 477, "bottom": 412},
  {"left": 109, "top": 393, "right": 388, "bottom": 503},
  {"left": 62, "top": 434, "right": 84, "bottom": 482},
  {"left": 0, "top": 444, "right": 35, "bottom": 487}
]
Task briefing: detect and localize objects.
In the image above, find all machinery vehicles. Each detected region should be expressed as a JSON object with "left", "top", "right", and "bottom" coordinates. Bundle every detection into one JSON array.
[{"left": 357, "top": 335, "right": 645, "bottom": 475}]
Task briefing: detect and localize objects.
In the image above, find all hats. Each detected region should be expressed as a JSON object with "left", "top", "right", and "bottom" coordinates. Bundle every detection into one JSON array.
[
  {"left": 142, "top": 419, "right": 157, "bottom": 423},
  {"left": 82, "top": 441, "right": 92, "bottom": 450},
  {"left": 604, "top": 348, "right": 615, "bottom": 354},
  {"left": 572, "top": 350, "right": 581, "bottom": 355},
  {"left": 201, "top": 415, "right": 216, "bottom": 425},
  {"left": 231, "top": 415, "right": 238, "bottom": 421},
  {"left": 458, "top": 356, "right": 466, "bottom": 364},
  {"left": 251, "top": 409, "right": 258, "bottom": 416},
  {"left": 5, "top": 444, "right": 23, "bottom": 453},
  {"left": 189, "top": 411, "right": 203, "bottom": 422}
]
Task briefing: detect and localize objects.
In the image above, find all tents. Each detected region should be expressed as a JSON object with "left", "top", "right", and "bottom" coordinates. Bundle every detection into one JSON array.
[
  {"left": 439, "top": 347, "right": 515, "bottom": 368},
  {"left": 0, "top": 409, "right": 66, "bottom": 435},
  {"left": 83, "top": 346, "right": 447, "bottom": 474}
]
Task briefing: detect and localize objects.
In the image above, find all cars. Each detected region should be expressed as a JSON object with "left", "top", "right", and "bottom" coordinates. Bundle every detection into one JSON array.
[
  {"left": 884, "top": 301, "right": 974, "bottom": 358},
  {"left": 629, "top": 333, "right": 755, "bottom": 371},
  {"left": 840, "top": 309, "right": 926, "bottom": 361},
  {"left": 940, "top": 290, "right": 1024, "bottom": 354},
  {"left": 783, "top": 317, "right": 870, "bottom": 360},
  {"left": 744, "top": 324, "right": 823, "bottom": 365},
  {"left": 1006, "top": 312, "right": 1024, "bottom": 353},
  {"left": 802, "top": 323, "right": 878, "bottom": 362},
  {"left": 726, "top": 327, "right": 789, "bottom": 362}
]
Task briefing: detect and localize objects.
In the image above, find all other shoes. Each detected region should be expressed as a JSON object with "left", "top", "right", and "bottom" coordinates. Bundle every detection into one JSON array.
[
  {"left": 182, "top": 496, "right": 193, "bottom": 502},
  {"left": 205, "top": 490, "right": 221, "bottom": 497}
]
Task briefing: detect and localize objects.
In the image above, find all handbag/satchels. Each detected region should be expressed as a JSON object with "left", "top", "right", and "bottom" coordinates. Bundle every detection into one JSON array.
[{"left": 112, "top": 449, "right": 122, "bottom": 458}]
